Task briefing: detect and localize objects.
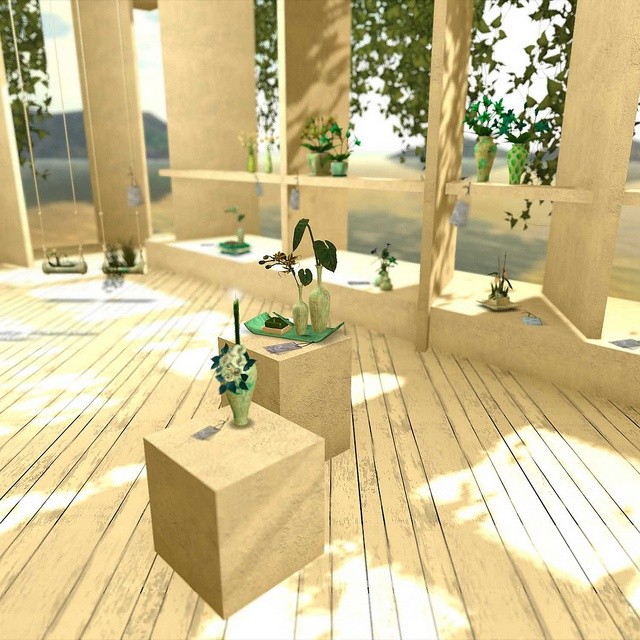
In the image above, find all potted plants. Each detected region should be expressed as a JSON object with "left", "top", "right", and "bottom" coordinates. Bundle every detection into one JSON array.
[
  {"left": 461, "top": 93, "right": 512, "bottom": 182},
  {"left": 370, "top": 242, "right": 391, "bottom": 285},
  {"left": 479, "top": 254, "right": 521, "bottom": 312},
  {"left": 239, "top": 129, "right": 257, "bottom": 171},
  {"left": 300, "top": 110, "right": 336, "bottom": 177},
  {"left": 259, "top": 250, "right": 312, "bottom": 336},
  {"left": 120, "top": 238, "right": 137, "bottom": 268},
  {"left": 293, "top": 215, "right": 338, "bottom": 333},
  {"left": 225, "top": 207, "right": 245, "bottom": 243},
  {"left": 321, "top": 122, "right": 361, "bottom": 176},
  {"left": 507, "top": 119, "right": 551, "bottom": 183},
  {"left": 262, "top": 136, "right": 280, "bottom": 174},
  {"left": 381, "top": 257, "right": 393, "bottom": 290}
]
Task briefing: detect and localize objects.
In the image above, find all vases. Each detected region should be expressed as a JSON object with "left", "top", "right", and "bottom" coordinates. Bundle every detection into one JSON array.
[{"left": 224, "top": 364, "right": 257, "bottom": 426}]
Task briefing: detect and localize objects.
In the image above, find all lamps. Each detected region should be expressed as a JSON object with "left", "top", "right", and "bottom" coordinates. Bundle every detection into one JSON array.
[{"left": 127, "top": 168, "right": 145, "bottom": 263}]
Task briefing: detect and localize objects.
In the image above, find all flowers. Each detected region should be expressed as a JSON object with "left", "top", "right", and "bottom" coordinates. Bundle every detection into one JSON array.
[{"left": 211, "top": 342, "right": 257, "bottom": 395}]
